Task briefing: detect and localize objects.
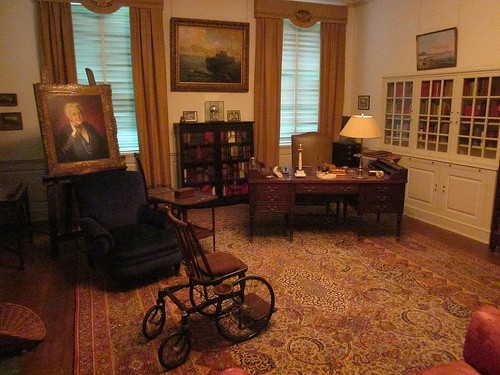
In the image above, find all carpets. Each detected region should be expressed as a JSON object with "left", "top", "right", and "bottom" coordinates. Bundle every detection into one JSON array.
[{"left": 74, "top": 204, "right": 500, "bottom": 375}]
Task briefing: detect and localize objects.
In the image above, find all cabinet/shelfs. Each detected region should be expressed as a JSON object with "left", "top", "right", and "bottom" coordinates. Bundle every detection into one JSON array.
[
  {"left": 380, "top": 66, "right": 500, "bottom": 244},
  {"left": 333, "top": 143, "right": 361, "bottom": 168},
  {"left": 173, "top": 121, "right": 256, "bottom": 210}
]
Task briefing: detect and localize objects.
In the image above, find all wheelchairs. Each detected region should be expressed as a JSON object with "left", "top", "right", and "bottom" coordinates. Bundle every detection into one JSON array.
[{"left": 140, "top": 206, "right": 278, "bottom": 370}]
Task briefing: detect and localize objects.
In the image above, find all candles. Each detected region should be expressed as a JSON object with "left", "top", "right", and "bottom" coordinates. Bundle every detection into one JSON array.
[{"left": 299, "top": 143, "right": 303, "bottom": 170}]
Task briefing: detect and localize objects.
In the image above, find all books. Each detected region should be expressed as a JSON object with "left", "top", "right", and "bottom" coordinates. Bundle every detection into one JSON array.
[
  {"left": 384, "top": 78, "right": 500, "bottom": 159},
  {"left": 222, "top": 162, "right": 248, "bottom": 179},
  {"left": 183, "top": 131, "right": 215, "bottom": 145},
  {"left": 184, "top": 146, "right": 214, "bottom": 162},
  {"left": 223, "top": 180, "right": 248, "bottom": 196},
  {"left": 221, "top": 145, "right": 250, "bottom": 161},
  {"left": 184, "top": 164, "right": 214, "bottom": 184},
  {"left": 221, "top": 130, "right": 250, "bottom": 143}
]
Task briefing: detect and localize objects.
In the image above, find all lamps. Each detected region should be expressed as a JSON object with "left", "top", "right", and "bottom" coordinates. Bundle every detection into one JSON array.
[{"left": 340, "top": 112, "right": 380, "bottom": 178}]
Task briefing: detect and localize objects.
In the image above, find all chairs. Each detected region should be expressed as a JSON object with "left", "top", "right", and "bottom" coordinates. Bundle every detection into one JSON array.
[{"left": 291, "top": 131, "right": 341, "bottom": 221}]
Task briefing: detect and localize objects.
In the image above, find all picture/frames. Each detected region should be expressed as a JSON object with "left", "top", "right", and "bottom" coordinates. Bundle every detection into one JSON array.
[
  {"left": 33, "top": 82, "right": 126, "bottom": 177},
  {"left": 204, "top": 101, "right": 224, "bottom": 121},
  {"left": 183, "top": 111, "right": 198, "bottom": 122},
  {"left": 357, "top": 95, "right": 370, "bottom": 110},
  {"left": 0, "top": 112, "right": 23, "bottom": 131},
  {"left": 0, "top": 93, "right": 18, "bottom": 107},
  {"left": 227, "top": 110, "right": 241, "bottom": 122},
  {"left": 416, "top": 26, "right": 457, "bottom": 72},
  {"left": 169, "top": 16, "right": 250, "bottom": 93}
]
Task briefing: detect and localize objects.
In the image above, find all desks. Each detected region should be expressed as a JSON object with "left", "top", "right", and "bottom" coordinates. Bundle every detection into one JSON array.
[
  {"left": 149, "top": 187, "right": 215, "bottom": 253},
  {"left": 247, "top": 166, "right": 409, "bottom": 243},
  {"left": 0, "top": 182, "right": 31, "bottom": 270}
]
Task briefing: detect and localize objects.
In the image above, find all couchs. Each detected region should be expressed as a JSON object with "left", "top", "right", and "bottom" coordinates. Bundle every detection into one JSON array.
[{"left": 58, "top": 170, "right": 182, "bottom": 291}]
